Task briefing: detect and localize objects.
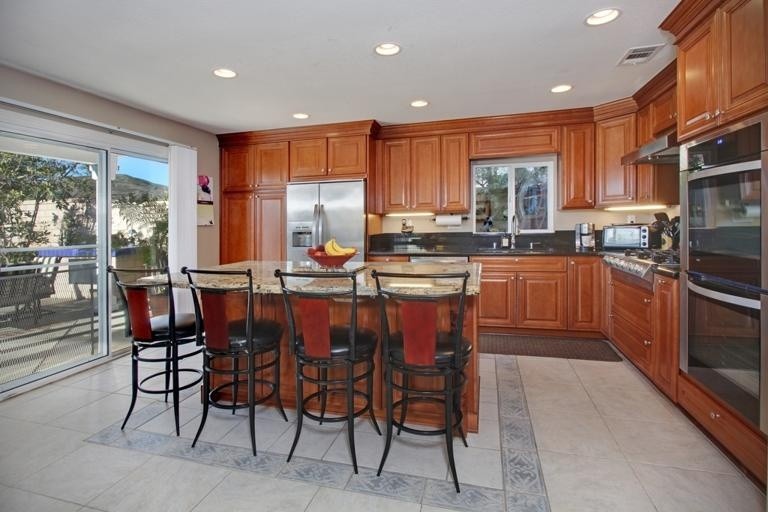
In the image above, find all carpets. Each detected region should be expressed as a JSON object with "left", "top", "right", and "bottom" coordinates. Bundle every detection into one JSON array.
[{"left": 477, "top": 333, "right": 623, "bottom": 362}]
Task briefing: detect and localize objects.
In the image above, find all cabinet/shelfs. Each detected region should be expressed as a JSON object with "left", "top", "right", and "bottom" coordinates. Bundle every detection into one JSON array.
[
  {"left": 678, "top": 374, "right": 767, "bottom": 495},
  {"left": 289, "top": 118, "right": 382, "bottom": 183},
  {"left": 654, "top": 273, "right": 680, "bottom": 403},
  {"left": 568, "top": 255, "right": 602, "bottom": 337},
  {"left": 384, "top": 119, "right": 440, "bottom": 213},
  {"left": 602, "top": 256, "right": 653, "bottom": 382},
  {"left": 632, "top": 87, "right": 679, "bottom": 206},
  {"left": 470, "top": 256, "right": 568, "bottom": 337},
  {"left": 219, "top": 192, "right": 286, "bottom": 266},
  {"left": 657, "top": 1, "right": 767, "bottom": 144},
  {"left": 650, "top": 85, "right": 678, "bottom": 138},
  {"left": 216, "top": 127, "right": 289, "bottom": 192},
  {"left": 596, "top": 96, "right": 637, "bottom": 210},
  {"left": 367, "top": 256, "right": 409, "bottom": 261},
  {"left": 440, "top": 116, "right": 474, "bottom": 215},
  {"left": 557, "top": 106, "right": 596, "bottom": 209}
]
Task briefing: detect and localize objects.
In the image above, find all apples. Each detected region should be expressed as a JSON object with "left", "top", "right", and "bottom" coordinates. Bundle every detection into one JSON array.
[{"left": 306, "top": 245, "right": 326, "bottom": 256}]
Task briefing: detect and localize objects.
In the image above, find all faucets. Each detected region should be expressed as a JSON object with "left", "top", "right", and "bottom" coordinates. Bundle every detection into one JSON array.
[{"left": 511, "top": 215, "right": 521, "bottom": 249}]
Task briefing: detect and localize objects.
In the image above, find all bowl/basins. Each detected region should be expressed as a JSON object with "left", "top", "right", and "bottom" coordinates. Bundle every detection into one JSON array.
[{"left": 304, "top": 252, "right": 356, "bottom": 268}]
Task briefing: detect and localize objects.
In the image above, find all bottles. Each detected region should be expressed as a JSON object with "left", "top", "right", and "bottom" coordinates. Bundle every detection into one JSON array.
[{"left": 501, "top": 230, "right": 510, "bottom": 249}]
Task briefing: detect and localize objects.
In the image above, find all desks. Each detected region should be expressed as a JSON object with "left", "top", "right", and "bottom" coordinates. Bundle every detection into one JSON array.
[{"left": 135, "top": 258, "right": 483, "bottom": 434}]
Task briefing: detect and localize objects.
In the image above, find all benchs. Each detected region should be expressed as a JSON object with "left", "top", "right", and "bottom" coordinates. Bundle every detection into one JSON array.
[{"left": 0, "top": 261, "right": 58, "bottom": 324}]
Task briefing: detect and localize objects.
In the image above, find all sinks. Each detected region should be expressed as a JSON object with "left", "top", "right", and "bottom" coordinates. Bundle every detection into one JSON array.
[
  {"left": 479, "top": 249, "right": 504, "bottom": 255},
  {"left": 505, "top": 250, "right": 530, "bottom": 255}
]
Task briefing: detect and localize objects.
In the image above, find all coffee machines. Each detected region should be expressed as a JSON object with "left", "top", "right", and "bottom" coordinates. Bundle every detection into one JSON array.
[{"left": 575, "top": 222, "right": 595, "bottom": 249}]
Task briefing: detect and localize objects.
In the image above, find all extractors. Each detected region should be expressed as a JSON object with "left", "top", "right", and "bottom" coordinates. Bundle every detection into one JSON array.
[{"left": 621, "top": 127, "right": 680, "bottom": 165}]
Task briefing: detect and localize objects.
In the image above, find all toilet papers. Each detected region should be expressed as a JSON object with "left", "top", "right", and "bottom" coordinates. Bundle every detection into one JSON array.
[{"left": 435, "top": 215, "right": 462, "bottom": 226}]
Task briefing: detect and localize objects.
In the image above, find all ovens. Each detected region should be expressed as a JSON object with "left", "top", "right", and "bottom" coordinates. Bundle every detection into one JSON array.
[{"left": 679, "top": 112, "right": 768, "bottom": 438}]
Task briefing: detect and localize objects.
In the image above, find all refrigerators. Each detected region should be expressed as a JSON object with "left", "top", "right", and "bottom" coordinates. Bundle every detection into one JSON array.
[{"left": 286, "top": 180, "right": 366, "bottom": 262}]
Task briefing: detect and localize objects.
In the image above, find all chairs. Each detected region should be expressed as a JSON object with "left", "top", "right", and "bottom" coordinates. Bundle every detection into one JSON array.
[
  {"left": 105, "top": 265, "right": 203, "bottom": 437},
  {"left": 180, "top": 266, "right": 288, "bottom": 458},
  {"left": 370, "top": 269, "right": 475, "bottom": 494},
  {"left": 272, "top": 269, "right": 383, "bottom": 475}
]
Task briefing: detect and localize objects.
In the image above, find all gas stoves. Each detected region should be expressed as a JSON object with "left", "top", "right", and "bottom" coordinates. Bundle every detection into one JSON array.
[{"left": 602, "top": 249, "right": 679, "bottom": 291}]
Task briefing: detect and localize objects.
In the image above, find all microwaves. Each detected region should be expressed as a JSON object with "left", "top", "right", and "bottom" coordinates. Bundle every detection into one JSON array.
[{"left": 603, "top": 225, "right": 651, "bottom": 250}]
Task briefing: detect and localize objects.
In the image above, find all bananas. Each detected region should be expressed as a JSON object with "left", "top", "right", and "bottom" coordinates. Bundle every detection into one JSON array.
[{"left": 325, "top": 238, "right": 356, "bottom": 256}]
